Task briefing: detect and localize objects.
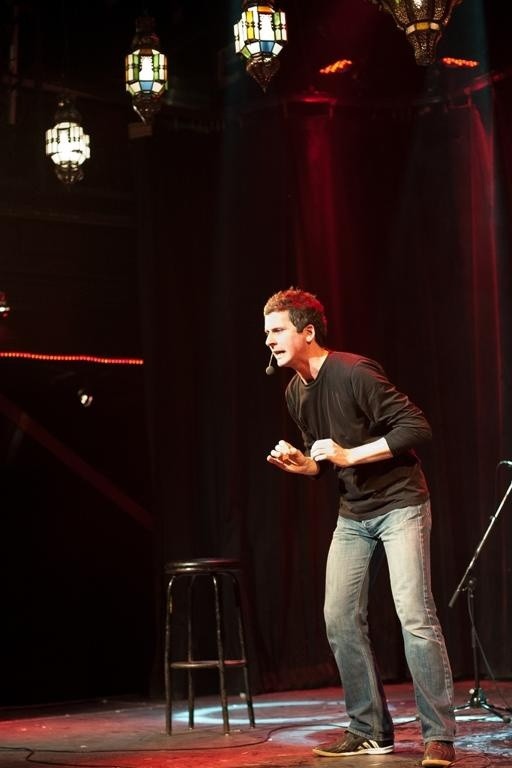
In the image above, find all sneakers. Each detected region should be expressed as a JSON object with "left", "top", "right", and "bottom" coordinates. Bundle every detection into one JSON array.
[
  {"left": 419, "top": 740, "right": 456, "bottom": 767},
  {"left": 311, "top": 726, "right": 396, "bottom": 759}
]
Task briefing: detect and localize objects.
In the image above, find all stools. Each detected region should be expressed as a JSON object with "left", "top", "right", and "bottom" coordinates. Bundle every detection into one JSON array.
[{"left": 164, "top": 557, "right": 256, "bottom": 735}]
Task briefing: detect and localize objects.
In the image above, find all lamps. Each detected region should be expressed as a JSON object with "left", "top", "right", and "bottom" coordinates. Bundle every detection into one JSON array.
[
  {"left": 123, "top": 27, "right": 170, "bottom": 125},
  {"left": 43, "top": 78, "right": 93, "bottom": 183},
  {"left": 232, "top": 2, "right": 290, "bottom": 96},
  {"left": 377, "top": 0, "right": 456, "bottom": 79}
]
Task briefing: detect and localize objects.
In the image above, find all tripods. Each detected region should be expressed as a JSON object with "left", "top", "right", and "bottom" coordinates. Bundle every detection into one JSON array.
[{"left": 452, "top": 578, "right": 512, "bottom": 724}]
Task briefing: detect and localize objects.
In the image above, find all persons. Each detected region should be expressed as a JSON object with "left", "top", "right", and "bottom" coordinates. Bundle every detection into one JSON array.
[{"left": 260, "top": 286, "right": 459, "bottom": 768}]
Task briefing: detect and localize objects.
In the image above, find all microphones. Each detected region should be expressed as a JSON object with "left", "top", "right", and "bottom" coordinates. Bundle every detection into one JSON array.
[{"left": 265, "top": 352, "right": 275, "bottom": 376}]
